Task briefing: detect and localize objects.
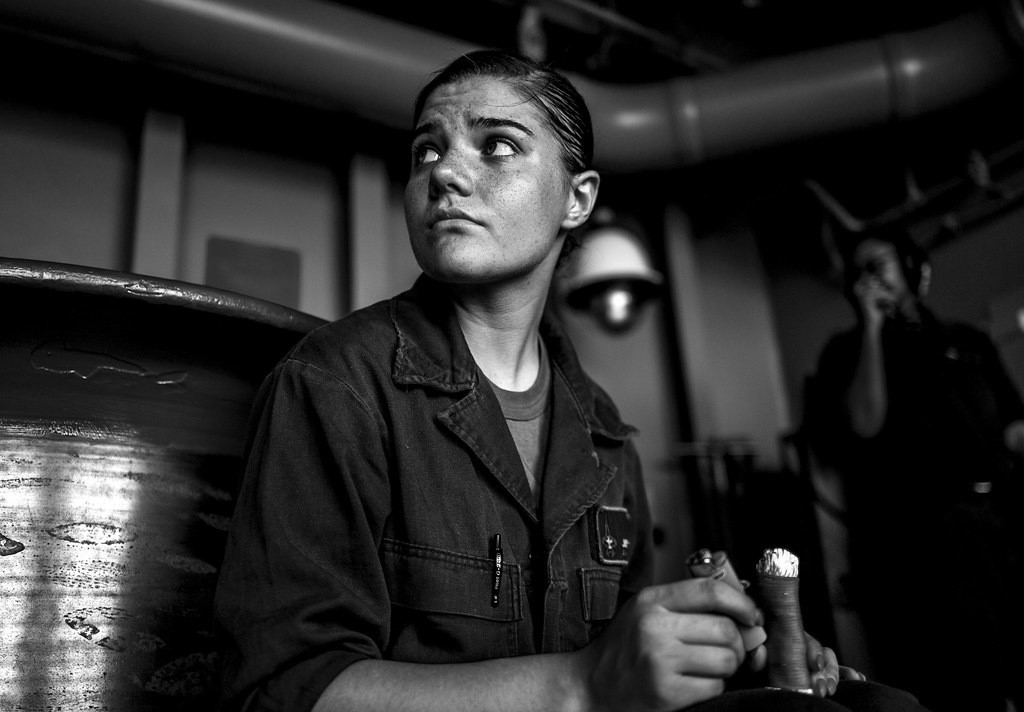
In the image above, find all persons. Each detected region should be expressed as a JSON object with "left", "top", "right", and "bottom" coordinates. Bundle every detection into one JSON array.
[
  {"left": 214, "top": 51, "right": 925, "bottom": 712},
  {"left": 815, "top": 223, "right": 1024, "bottom": 712}
]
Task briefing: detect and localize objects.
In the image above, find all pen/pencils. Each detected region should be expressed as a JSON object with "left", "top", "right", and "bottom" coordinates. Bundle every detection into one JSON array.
[{"left": 491, "top": 533, "right": 504, "bottom": 607}]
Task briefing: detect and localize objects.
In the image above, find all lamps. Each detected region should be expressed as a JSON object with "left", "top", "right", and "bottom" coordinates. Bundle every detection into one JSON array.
[{"left": 557, "top": 232, "right": 662, "bottom": 335}]
